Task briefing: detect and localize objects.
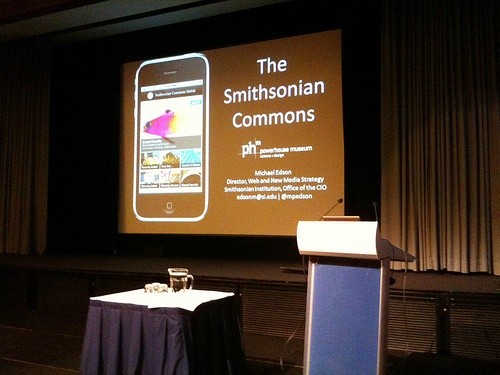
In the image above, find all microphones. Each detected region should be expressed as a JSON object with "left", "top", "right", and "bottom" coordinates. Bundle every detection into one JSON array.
[
  {"left": 373, "top": 202, "right": 378, "bottom": 221},
  {"left": 319, "top": 199, "right": 343, "bottom": 221}
]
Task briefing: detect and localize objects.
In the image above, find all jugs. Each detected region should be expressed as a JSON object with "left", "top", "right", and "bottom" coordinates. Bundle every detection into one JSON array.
[{"left": 168, "top": 268, "right": 194, "bottom": 295}]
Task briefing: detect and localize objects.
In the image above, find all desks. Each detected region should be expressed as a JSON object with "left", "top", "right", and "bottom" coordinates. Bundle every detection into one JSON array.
[{"left": 80, "top": 288, "right": 247, "bottom": 375}]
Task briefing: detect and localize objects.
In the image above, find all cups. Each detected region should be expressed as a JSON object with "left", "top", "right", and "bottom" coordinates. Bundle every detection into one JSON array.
[{"left": 145, "top": 283, "right": 167, "bottom": 293}]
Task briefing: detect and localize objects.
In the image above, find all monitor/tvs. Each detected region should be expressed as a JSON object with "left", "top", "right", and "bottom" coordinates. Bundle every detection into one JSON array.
[{"left": 323, "top": 216, "right": 360, "bottom": 221}]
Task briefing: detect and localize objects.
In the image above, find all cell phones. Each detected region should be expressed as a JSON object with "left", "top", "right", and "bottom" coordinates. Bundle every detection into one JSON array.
[{"left": 132, "top": 52, "right": 209, "bottom": 223}]
